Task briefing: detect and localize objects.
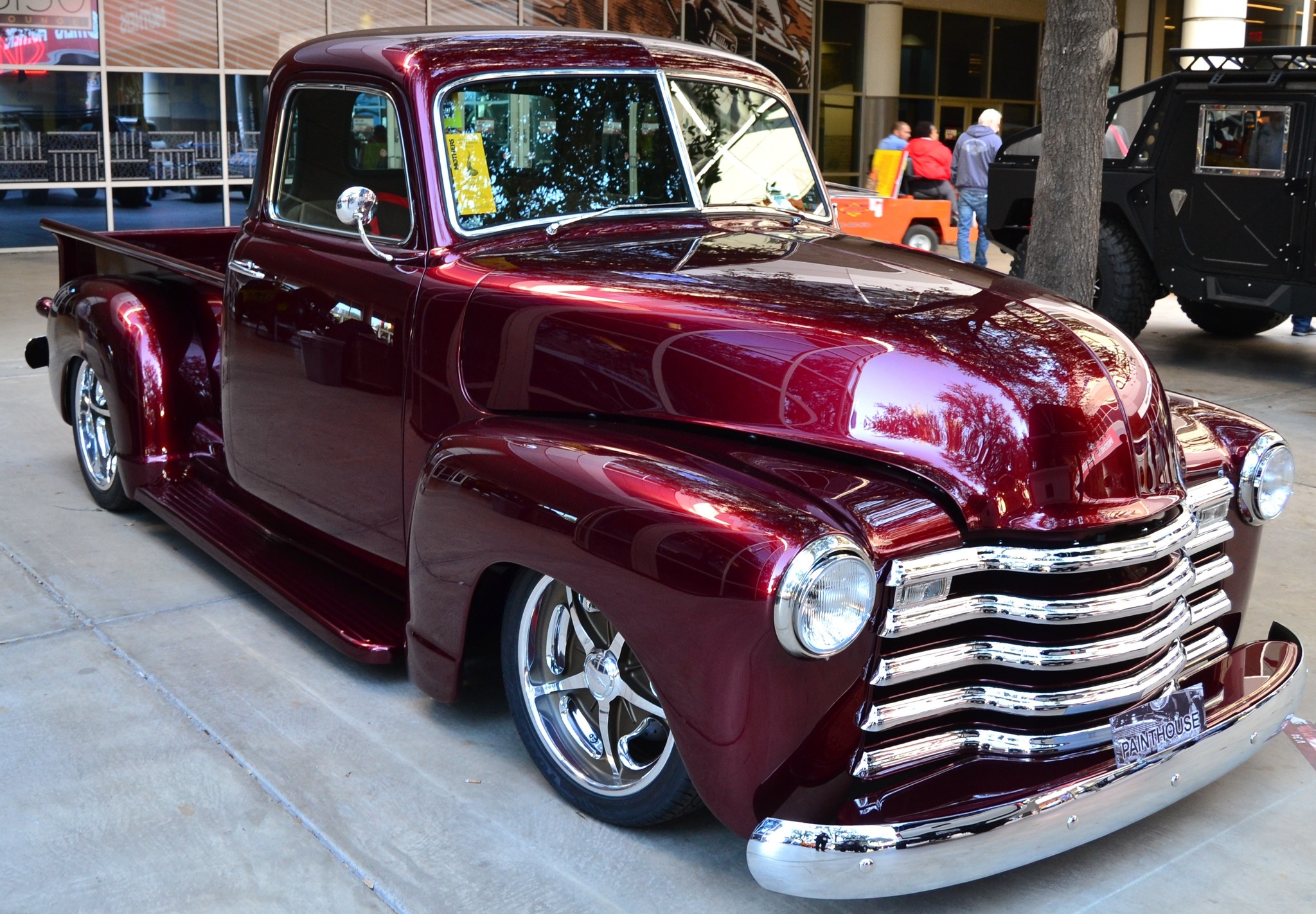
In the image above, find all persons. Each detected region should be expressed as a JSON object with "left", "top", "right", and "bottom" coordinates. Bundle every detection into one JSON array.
[
  {"left": 949, "top": 108, "right": 1004, "bottom": 266},
  {"left": 877, "top": 121, "right": 912, "bottom": 151},
  {"left": 903, "top": 124, "right": 959, "bottom": 227},
  {"left": 1291, "top": 314, "right": 1316, "bottom": 337}
]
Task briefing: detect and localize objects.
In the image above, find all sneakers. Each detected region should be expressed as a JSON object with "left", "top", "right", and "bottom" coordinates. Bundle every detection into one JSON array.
[{"left": 1291, "top": 326, "right": 1316, "bottom": 336}]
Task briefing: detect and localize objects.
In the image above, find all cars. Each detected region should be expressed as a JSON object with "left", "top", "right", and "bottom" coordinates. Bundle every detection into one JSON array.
[{"left": 0, "top": 91, "right": 258, "bottom": 206}]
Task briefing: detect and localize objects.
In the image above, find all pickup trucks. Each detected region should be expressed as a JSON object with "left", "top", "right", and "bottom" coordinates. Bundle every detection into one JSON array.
[
  {"left": 988, "top": 46, "right": 1314, "bottom": 349},
  {"left": 21, "top": 24, "right": 1302, "bottom": 901}
]
